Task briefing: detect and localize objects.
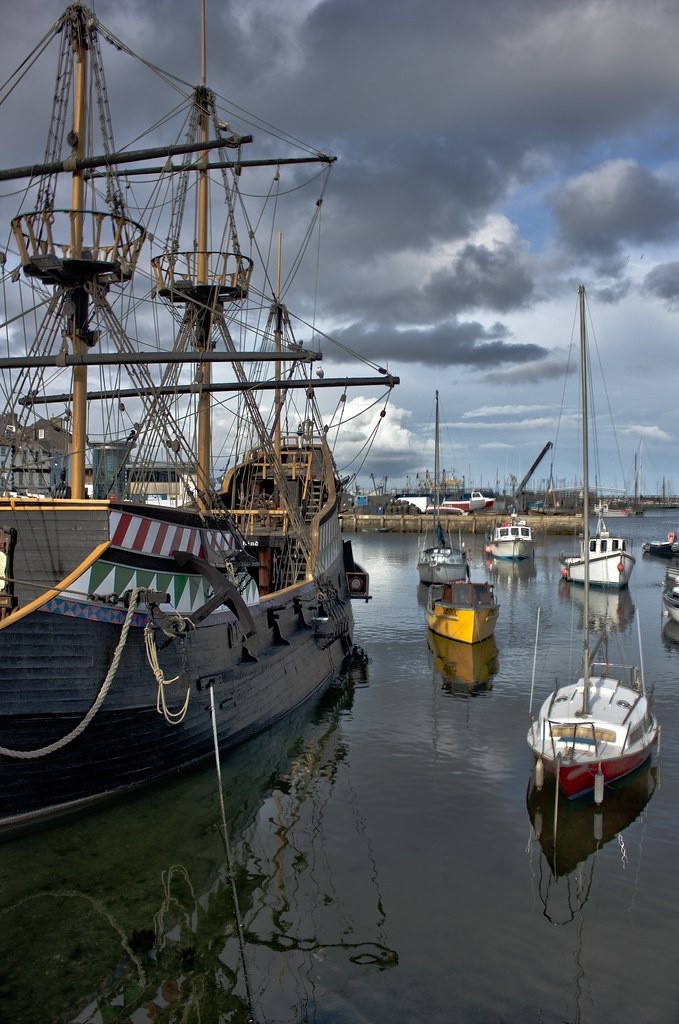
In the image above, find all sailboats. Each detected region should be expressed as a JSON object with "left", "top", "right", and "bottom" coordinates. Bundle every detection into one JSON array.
[
  {"left": 0, "top": 4, "right": 401, "bottom": 817},
  {"left": 562, "top": 578, "right": 637, "bottom": 673},
  {"left": 416, "top": 388, "right": 468, "bottom": 581},
  {"left": 523, "top": 284, "right": 663, "bottom": 798}
]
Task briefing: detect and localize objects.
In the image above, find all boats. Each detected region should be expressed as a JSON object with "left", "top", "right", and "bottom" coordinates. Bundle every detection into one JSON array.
[
  {"left": 593, "top": 502, "right": 632, "bottom": 519},
  {"left": 426, "top": 579, "right": 501, "bottom": 645},
  {"left": 425, "top": 626, "right": 499, "bottom": 698},
  {"left": 444, "top": 491, "right": 494, "bottom": 515},
  {"left": 661, "top": 565, "right": 679, "bottom": 623},
  {"left": 484, "top": 511, "right": 537, "bottom": 561},
  {"left": 640, "top": 531, "right": 679, "bottom": 556},
  {"left": 558, "top": 496, "right": 636, "bottom": 588},
  {"left": 525, "top": 762, "right": 659, "bottom": 924}
]
